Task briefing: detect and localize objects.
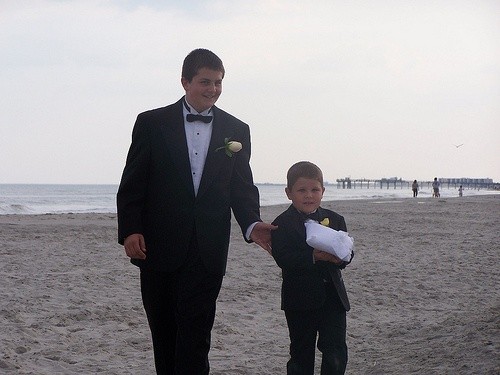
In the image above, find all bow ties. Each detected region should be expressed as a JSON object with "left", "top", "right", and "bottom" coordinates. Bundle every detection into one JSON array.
[
  {"left": 301, "top": 211, "right": 318, "bottom": 221},
  {"left": 184, "top": 99, "right": 212, "bottom": 123}
]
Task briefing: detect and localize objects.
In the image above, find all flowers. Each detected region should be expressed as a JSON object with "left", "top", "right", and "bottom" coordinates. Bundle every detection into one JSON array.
[
  {"left": 319, "top": 217, "right": 329, "bottom": 226},
  {"left": 214, "top": 137, "right": 242, "bottom": 157}
]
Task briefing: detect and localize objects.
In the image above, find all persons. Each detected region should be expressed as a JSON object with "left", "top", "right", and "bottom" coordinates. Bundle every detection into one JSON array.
[
  {"left": 459, "top": 185, "right": 464, "bottom": 196},
  {"left": 270, "top": 162, "right": 355, "bottom": 375},
  {"left": 432, "top": 177, "right": 440, "bottom": 197},
  {"left": 412, "top": 180, "right": 419, "bottom": 197},
  {"left": 117, "top": 49, "right": 277, "bottom": 375}
]
